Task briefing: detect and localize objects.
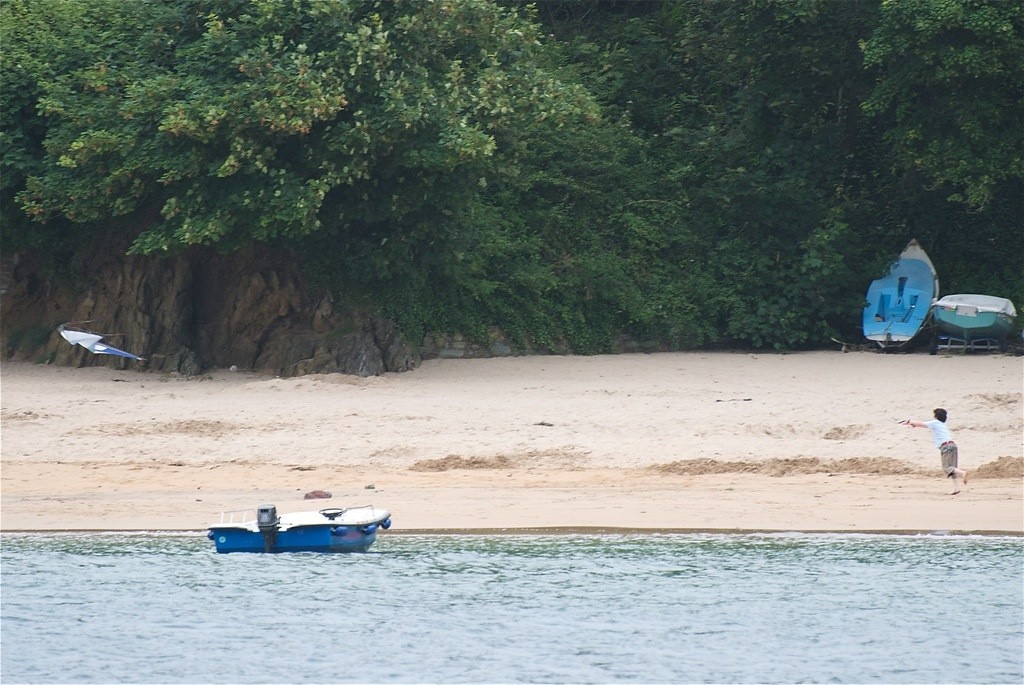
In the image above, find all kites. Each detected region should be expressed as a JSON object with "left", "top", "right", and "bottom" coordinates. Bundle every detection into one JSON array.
[{"left": 57, "top": 321, "right": 146, "bottom": 361}]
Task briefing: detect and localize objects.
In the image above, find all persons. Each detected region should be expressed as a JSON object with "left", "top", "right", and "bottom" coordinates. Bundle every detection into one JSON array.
[{"left": 899, "top": 408, "right": 969, "bottom": 495}]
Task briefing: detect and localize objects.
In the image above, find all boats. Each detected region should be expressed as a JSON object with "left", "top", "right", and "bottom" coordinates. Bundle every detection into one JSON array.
[
  {"left": 861, "top": 236, "right": 940, "bottom": 354},
  {"left": 932, "top": 293, "right": 1020, "bottom": 354},
  {"left": 207, "top": 502, "right": 391, "bottom": 554}
]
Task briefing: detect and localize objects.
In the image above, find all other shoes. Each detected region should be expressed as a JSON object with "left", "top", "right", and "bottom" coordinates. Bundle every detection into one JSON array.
[
  {"left": 945, "top": 488, "right": 960, "bottom": 496},
  {"left": 962, "top": 471, "right": 968, "bottom": 485}
]
